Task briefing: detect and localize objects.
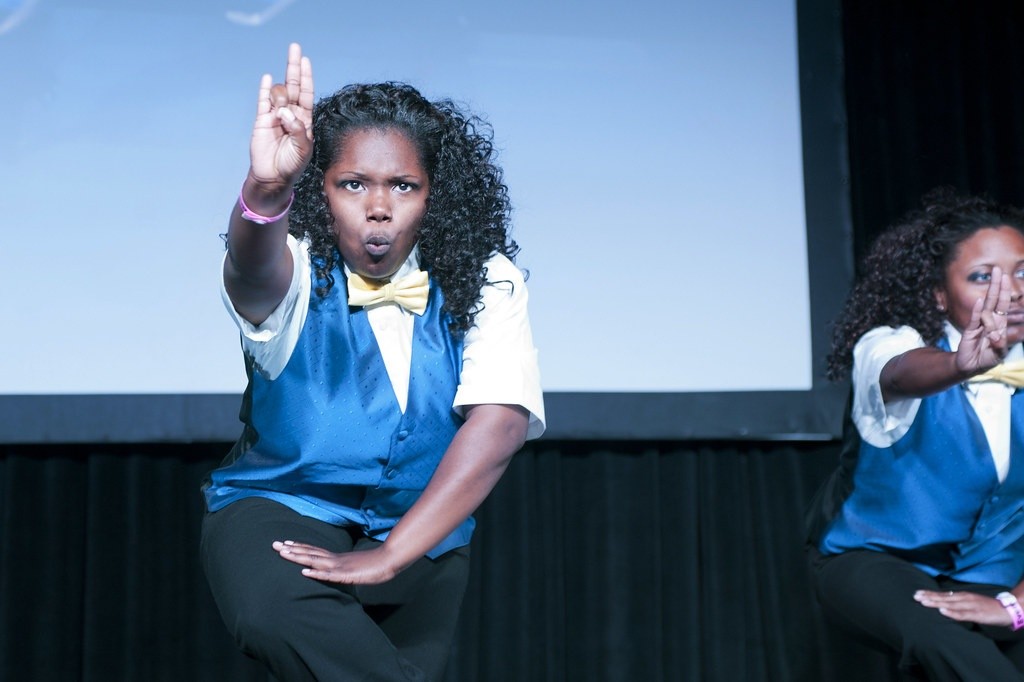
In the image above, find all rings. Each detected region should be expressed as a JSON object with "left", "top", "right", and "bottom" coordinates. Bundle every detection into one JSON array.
[
  {"left": 949, "top": 592, "right": 953, "bottom": 595},
  {"left": 995, "top": 309, "right": 1008, "bottom": 315}
]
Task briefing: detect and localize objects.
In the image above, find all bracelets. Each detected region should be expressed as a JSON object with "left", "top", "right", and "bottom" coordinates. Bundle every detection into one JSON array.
[
  {"left": 239, "top": 179, "right": 294, "bottom": 223},
  {"left": 996, "top": 592, "right": 1024, "bottom": 631}
]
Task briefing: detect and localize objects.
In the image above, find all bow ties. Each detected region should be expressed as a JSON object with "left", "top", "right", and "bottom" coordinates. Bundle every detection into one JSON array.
[
  {"left": 952, "top": 338, "right": 1024, "bottom": 387},
  {"left": 347, "top": 269, "right": 429, "bottom": 316}
]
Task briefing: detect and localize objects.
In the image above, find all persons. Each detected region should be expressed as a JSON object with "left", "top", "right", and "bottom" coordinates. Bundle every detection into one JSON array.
[
  {"left": 202, "top": 43, "right": 546, "bottom": 682},
  {"left": 813, "top": 199, "right": 1024, "bottom": 682}
]
